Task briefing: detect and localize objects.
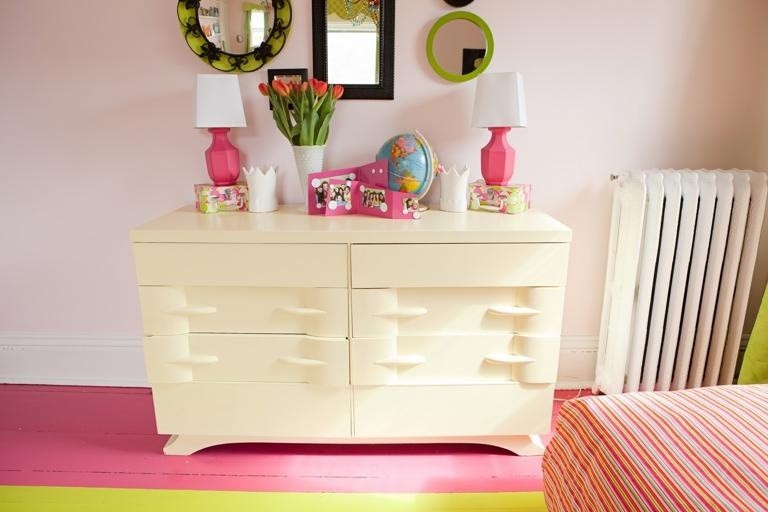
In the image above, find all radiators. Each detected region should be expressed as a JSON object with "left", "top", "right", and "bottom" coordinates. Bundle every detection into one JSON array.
[{"left": 592, "top": 169, "right": 766, "bottom": 394}]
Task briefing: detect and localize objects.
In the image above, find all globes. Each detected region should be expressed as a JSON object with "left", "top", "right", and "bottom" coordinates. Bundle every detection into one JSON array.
[{"left": 375, "top": 130, "right": 438, "bottom": 202}]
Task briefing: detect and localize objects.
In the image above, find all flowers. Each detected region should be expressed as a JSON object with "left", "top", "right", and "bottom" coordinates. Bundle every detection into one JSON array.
[{"left": 258, "top": 79, "right": 344, "bottom": 145}]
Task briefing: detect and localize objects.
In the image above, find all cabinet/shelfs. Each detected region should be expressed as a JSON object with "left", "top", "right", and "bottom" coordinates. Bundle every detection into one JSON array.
[{"left": 129, "top": 204, "right": 571, "bottom": 457}]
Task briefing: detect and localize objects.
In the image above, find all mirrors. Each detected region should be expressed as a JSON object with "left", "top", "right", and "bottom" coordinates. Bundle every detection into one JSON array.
[
  {"left": 176, "top": 0, "right": 293, "bottom": 73},
  {"left": 313, "top": 0, "right": 396, "bottom": 101},
  {"left": 427, "top": 11, "right": 498, "bottom": 84}
]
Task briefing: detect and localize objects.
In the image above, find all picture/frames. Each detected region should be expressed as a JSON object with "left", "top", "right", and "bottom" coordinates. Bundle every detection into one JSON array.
[
  {"left": 306, "top": 159, "right": 389, "bottom": 215},
  {"left": 323, "top": 179, "right": 419, "bottom": 219},
  {"left": 267, "top": 69, "right": 308, "bottom": 109}
]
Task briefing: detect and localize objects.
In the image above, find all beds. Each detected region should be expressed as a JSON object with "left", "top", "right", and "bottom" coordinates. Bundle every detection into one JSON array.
[{"left": 543, "top": 387, "right": 768, "bottom": 512}]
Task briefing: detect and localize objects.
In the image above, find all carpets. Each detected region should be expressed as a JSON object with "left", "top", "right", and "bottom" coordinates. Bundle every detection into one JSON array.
[{"left": 1, "top": 487, "right": 547, "bottom": 512}]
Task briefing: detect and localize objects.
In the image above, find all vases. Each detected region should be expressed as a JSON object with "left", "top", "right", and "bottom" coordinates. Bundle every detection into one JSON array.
[{"left": 293, "top": 145, "right": 324, "bottom": 210}]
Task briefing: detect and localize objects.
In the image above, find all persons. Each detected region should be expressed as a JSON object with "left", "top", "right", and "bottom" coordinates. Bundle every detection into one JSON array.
[{"left": 314, "top": 178, "right": 416, "bottom": 212}]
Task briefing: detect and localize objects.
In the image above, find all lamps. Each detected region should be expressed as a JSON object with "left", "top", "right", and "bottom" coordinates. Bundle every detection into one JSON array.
[
  {"left": 471, "top": 72, "right": 528, "bottom": 187},
  {"left": 193, "top": 75, "right": 248, "bottom": 186}
]
